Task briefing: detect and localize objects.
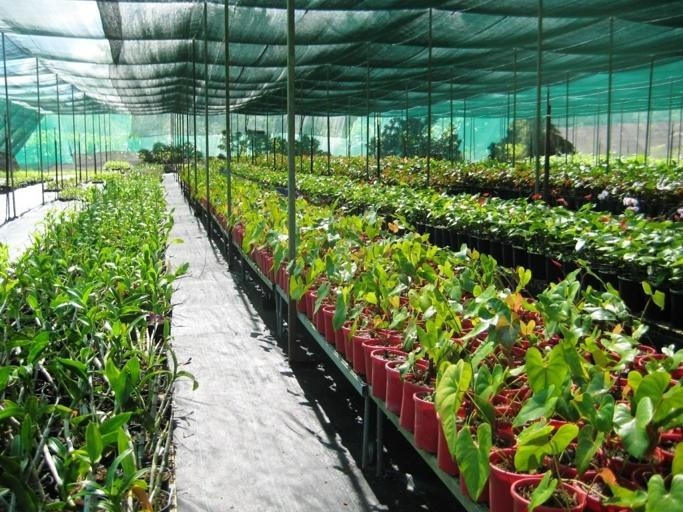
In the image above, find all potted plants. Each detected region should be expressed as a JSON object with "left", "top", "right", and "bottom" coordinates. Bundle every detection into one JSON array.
[{"left": 200, "top": 178, "right": 683, "bottom": 511}]
